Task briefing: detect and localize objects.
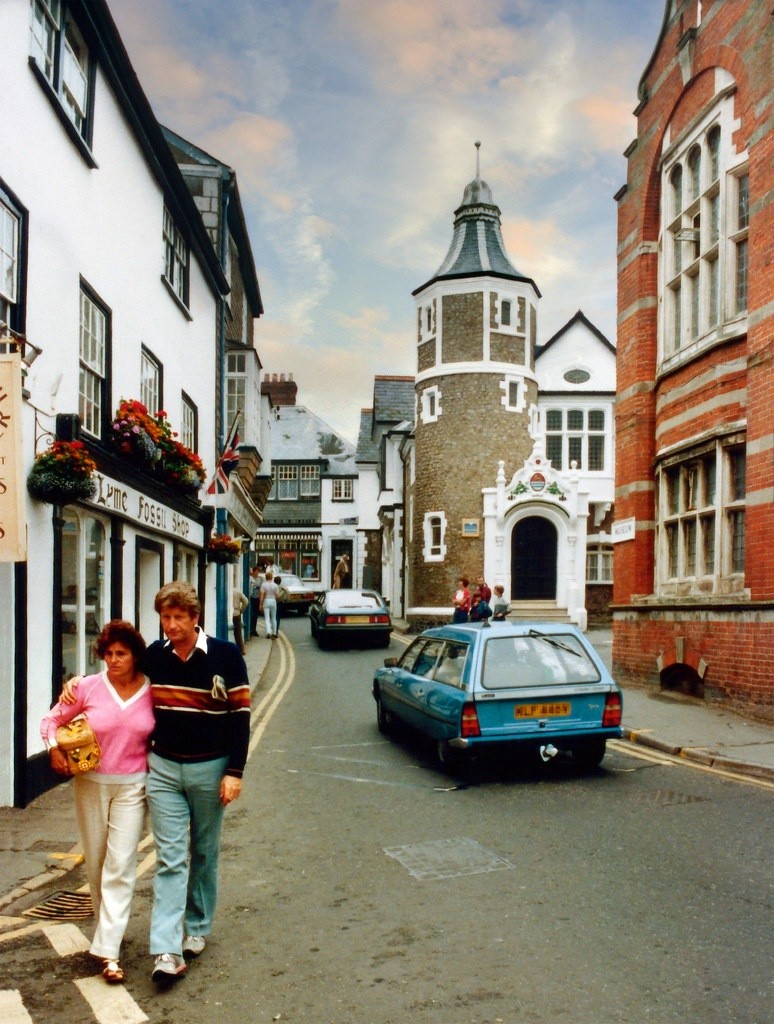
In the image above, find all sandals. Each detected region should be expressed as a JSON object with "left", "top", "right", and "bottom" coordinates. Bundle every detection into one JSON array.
[{"left": 100, "top": 957, "right": 125, "bottom": 982}]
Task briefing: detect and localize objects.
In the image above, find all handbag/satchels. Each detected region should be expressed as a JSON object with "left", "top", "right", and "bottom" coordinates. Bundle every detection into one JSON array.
[{"left": 57, "top": 718, "right": 102, "bottom": 774}]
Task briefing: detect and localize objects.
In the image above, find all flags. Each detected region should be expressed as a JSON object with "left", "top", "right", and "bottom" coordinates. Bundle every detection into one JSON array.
[{"left": 207, "top": 423, "right": 240, "bottom": 494}]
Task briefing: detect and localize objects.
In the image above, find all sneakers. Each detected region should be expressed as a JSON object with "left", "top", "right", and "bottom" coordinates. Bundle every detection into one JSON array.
[
  {"left": 183, "top": 934, "right": 205, "bottom": 957},
  {"left": 152, "top": 952, "right": 186, "bottom": 981}
]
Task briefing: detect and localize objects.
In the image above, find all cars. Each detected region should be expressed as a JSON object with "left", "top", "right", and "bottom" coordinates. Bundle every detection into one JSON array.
[
  {"left": 371, "top": 619, "right": 624, "bottom": 773},
  {"left": 307, "top": 589, "right": 394, "bottom": 648},
  {"left": 256, "top": 573, "right": 319, "bottom": 616}
]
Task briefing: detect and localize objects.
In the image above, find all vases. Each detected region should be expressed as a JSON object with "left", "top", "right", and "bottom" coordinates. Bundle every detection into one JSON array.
[
  {"left": 35, "top": 487, "right": 85, "bottom": 505},
  {"left": 210, "top": 551, "right": 234, "bottom": 566}
]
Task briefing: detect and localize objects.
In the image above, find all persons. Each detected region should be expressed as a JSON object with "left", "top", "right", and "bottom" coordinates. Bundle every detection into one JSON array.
[
  {"left": 423, "top": 643, "right": 467, "bottom": 687},
  {"left": 469, "top": 590, "right": 486, "bottom": 622},
  {"left": 471, "top": 574, "right": 491, "bottom": 605},
  {"left": 58, "top": 580, "right": 252, "bottom": 976},
  {"left": 311, "top": 570, "right": 318, "bottom": 578},
  {"left": 249, "top": 562, "right": 263, "bottom": 637},
  {"left": 274, "top": 576, "right": 288, "bottom": 637},
  {"left": 41, "top": 619, "right": 154, "bottom": 984},
  {"left": 448, "top": 576, "right": 472, "bottom": 623},
  {"left": 333, "top": 554, "right": 348, "bottom": 589},
  {"left": 491, "top": 584, "right": 514, "bottom": 622},
  {"left": 233, "top": 585, "right": 248, "bottom": 657},
  {"left": 266, "top": 560, "right": 279, "bottom": 573},
  {"left": 258, "top": 571, "right": 281, "bottom": 639}
]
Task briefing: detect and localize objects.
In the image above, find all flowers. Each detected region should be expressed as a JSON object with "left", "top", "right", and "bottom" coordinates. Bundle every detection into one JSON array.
[
  {"left": 115, "top": 398, "right": 206, "bottom": 494},
  {"left": 209, "top": 532, "right": 252, "bottom": 562},
  {"left": 30, "top": 442, "right": 93, "bottom": 493}
]
